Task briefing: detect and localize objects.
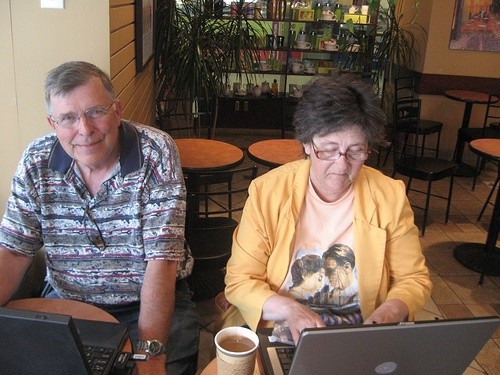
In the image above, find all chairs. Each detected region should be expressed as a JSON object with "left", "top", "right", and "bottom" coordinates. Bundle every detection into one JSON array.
[{"left": 154, "top": 74, "right": 500, "bottom": 290}]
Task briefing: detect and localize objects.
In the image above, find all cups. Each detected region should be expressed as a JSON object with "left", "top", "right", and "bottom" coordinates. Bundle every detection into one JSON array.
[
  {"left": 243, "top": 83, "right": 254, "bottom": 93},
  {"left": 212, "top": 327, "right": 259, "bottom": 375},
  {"left": 252, "top": 87, "right": 263, "bottom": 96},
  {"left": 239, "top": 90, "right": 243, "bottom": 94},
  {"left": 276, "top": 36, "right": 285, "bottom": 49},
  {"left": 233, "top": 82, "right": 241, "bottom": 93},
  {"left": 290, "top": 60, "right": 318, "bottom": 72},
  {"left": 266, "top": 33, "right": 274, "bottom": 47},
  {"left": 295, "top": 90, "right": 303, "bottom": 96},
  {"left": 324, "top": 43, "right": 339, "bottom": 49},
  {"left": 296, "top": 41, "right": 311, "bottom": 47},
  {"left": 322, "top": 13, "right": 337, "bottom": 19}
]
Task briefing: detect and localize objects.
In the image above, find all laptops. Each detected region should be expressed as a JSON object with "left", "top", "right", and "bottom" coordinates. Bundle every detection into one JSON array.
[
  {"left": 0, "top": 308, "right": 130, "bottom": 375},
  {"left": 256, "top": 315, "right": 500, "bottom": 375}
]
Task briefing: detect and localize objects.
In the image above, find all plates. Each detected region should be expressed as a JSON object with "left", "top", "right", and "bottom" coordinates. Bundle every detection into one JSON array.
[
  {"left": 321, "top": 49, "right": 338, "bottom": 51},
  {"left": 320, "top": 19, "right": 337, "bottom": 22},
  {"left": 292, "top": 47, "right": 310, "bottom": 50},
  {"left": 235, "top": 93, "right": 246, "bottom": 96},
  {"left": 290, "top": 95, "right": 304, "bottom": 98}
]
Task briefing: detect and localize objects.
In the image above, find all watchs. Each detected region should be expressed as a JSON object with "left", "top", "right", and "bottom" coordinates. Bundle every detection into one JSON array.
[{"left": 136, "top": 340, "right": 165, "bottom": 357}]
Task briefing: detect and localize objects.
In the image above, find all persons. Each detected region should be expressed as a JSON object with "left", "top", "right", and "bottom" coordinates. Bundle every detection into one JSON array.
[
  {"left": 0, "top": 61, "right": 199, "bottom": 375},
  {"left": 221, "top": 71, "right": 432, "bottom": 347}
]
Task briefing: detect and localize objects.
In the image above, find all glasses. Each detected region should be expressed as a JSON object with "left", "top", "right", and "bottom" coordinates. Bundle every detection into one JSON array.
[
  {"left": 81, "top": 201, "right": 106, "bottom": 252},
  {"left": 310, "top": 139, "right": 370, "bottom": 162},
  {"left": 51, "top": 102, "right": 114, "bottom": 129}
]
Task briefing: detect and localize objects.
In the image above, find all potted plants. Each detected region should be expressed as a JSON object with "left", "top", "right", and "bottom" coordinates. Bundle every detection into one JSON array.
[
  {"left": 334, "top": 0, "right": 428, "bottom": 138},
  {"left": 152, "top": 0, "right": 276, "bottom": 139}
]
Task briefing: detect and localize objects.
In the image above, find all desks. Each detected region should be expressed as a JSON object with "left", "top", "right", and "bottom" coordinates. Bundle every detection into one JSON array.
[{"left": 3, "top": 89, "right": 500, "bottom": 375}]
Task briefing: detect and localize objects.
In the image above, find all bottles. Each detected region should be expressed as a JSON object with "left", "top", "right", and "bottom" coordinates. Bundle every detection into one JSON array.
[
  {"left": 271, "top": 79, "right": 279, "bottom": 97},
  {"left": 290, "top": 29, "right": 316, "bottom": 49},
  {"left": 314, "top": 3, "right": 341, "bottom": 22},
  {"left": 262, "top": 80, "right": 270, "bottom": 95}
]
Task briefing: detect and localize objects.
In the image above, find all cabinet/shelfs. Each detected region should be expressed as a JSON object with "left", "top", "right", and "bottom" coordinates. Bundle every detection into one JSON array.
[{"left": 195, "top": 0, "right": 381, "bottom": 129}]
